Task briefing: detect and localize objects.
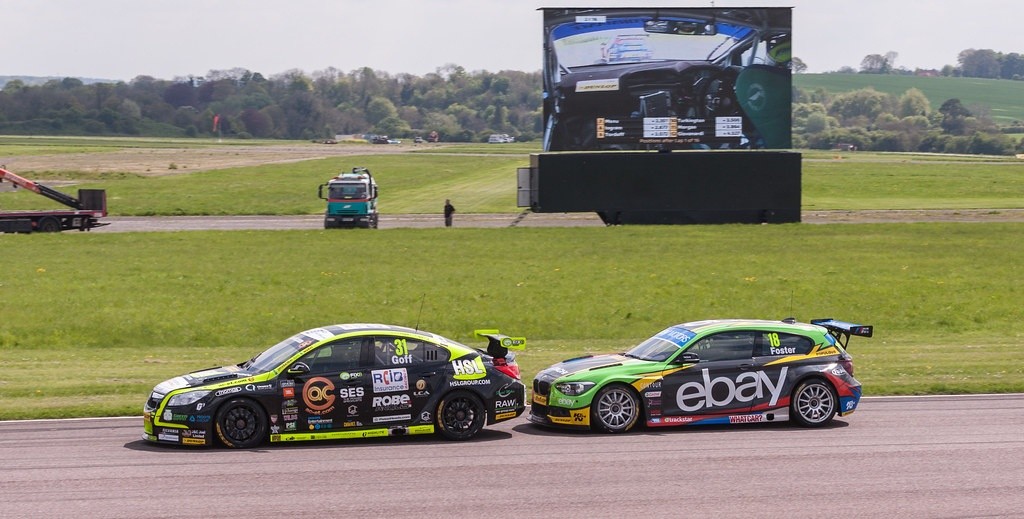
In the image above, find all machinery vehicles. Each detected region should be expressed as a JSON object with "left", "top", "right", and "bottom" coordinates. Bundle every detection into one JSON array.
[{"left": 0, "top": 168, "right": 112, "bottom": 234}]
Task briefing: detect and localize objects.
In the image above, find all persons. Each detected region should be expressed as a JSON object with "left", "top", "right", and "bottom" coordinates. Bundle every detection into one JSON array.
[{"left": 445, "top": 199, "right": 455, "bottom": 227}]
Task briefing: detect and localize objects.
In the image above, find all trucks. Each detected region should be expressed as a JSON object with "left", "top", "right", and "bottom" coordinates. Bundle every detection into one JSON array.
[
  {"left": 489, "top": 134, "right": 514, "bottom": 143},
  {"left": 428, "top": 131, "right": 439, "bottom": 142},
  {"left": 317, "top": 168, "right": 380, "bottom": 229}
]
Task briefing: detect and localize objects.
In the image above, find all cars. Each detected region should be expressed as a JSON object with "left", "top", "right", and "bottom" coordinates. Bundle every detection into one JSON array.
[
  {"left": 142, "top": 322, "right": 527, "bottom": 450},
  {"left": 526, "top": 318, "right": 873, "bottom": 434},
  {"left": 414, "top": 137, "right": 423, "bottom": 143}
]
